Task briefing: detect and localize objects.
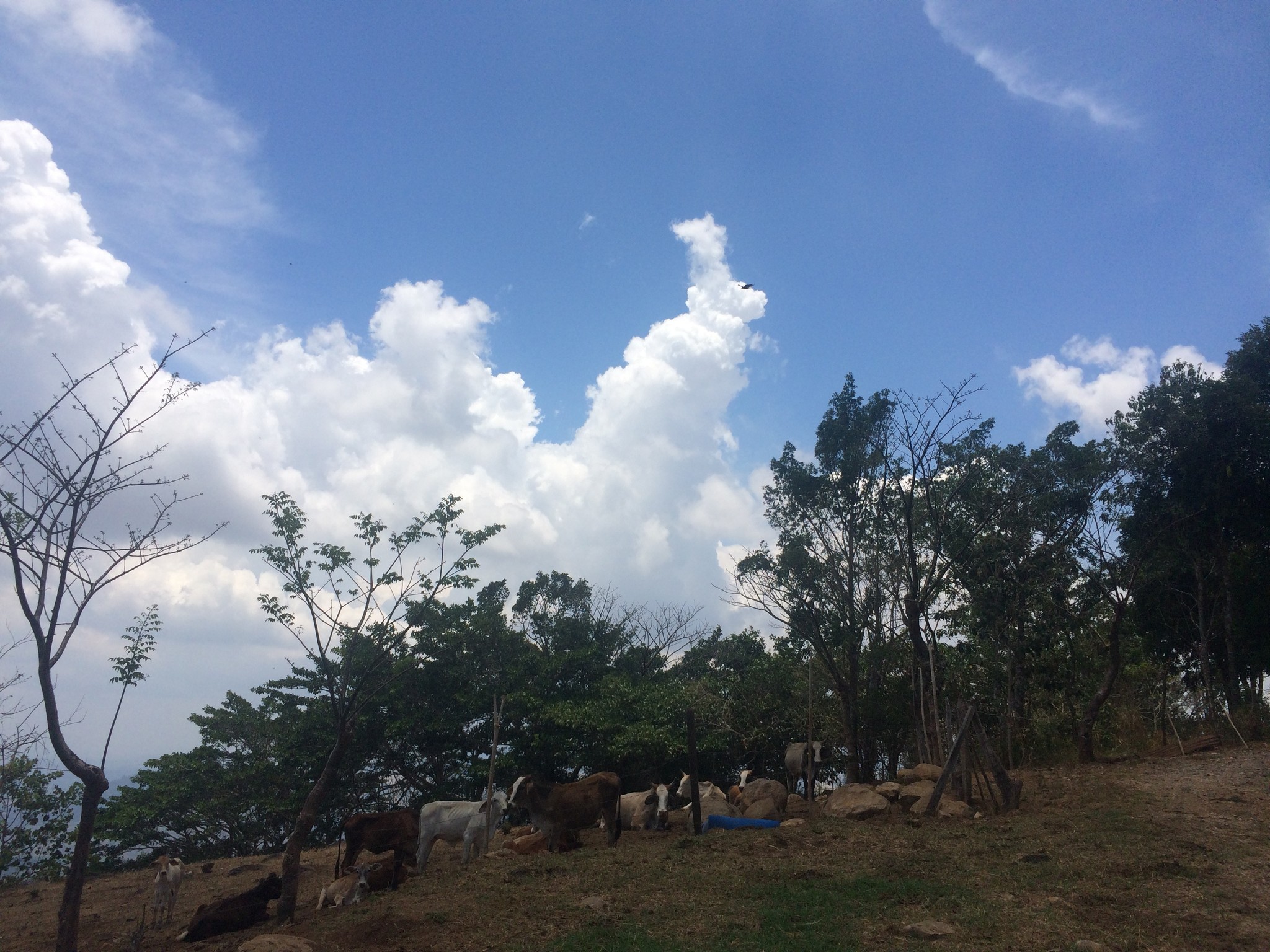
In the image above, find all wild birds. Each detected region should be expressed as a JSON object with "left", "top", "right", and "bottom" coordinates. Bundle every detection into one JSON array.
[{"left": 738, "top": 284, "right": 754, "bottom": 290}]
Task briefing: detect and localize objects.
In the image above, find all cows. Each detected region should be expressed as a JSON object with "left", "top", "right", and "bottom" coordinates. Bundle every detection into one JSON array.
[
  {"left": 600, "top": 777, "right": 677, "bottom": 833},
  {"left": 783, "top": 735, "right": 827, "bottom": 800},
  {"left": 333, "top": 810, "right": 420, "bottom": 891},
  {"left": 415, "top": 792, "right": 509, "bottom": 867},
  {"left": 315, "top": 861, "right": 383, "bottom": 909},
  {"left": 728, "top": 763, "right": 758, "bottom": 805},
  {"left": 677, "top": 768, "right": 727, "bottom": 811},
  {"left": 509, "top": 770, "right": 620, "bottom": 854},
  {"left": 149, "top": 854, "right": 185, "bottom": 926},
  {"left": 175, "top": 871, "right": 284, "bottom": 943}
]
